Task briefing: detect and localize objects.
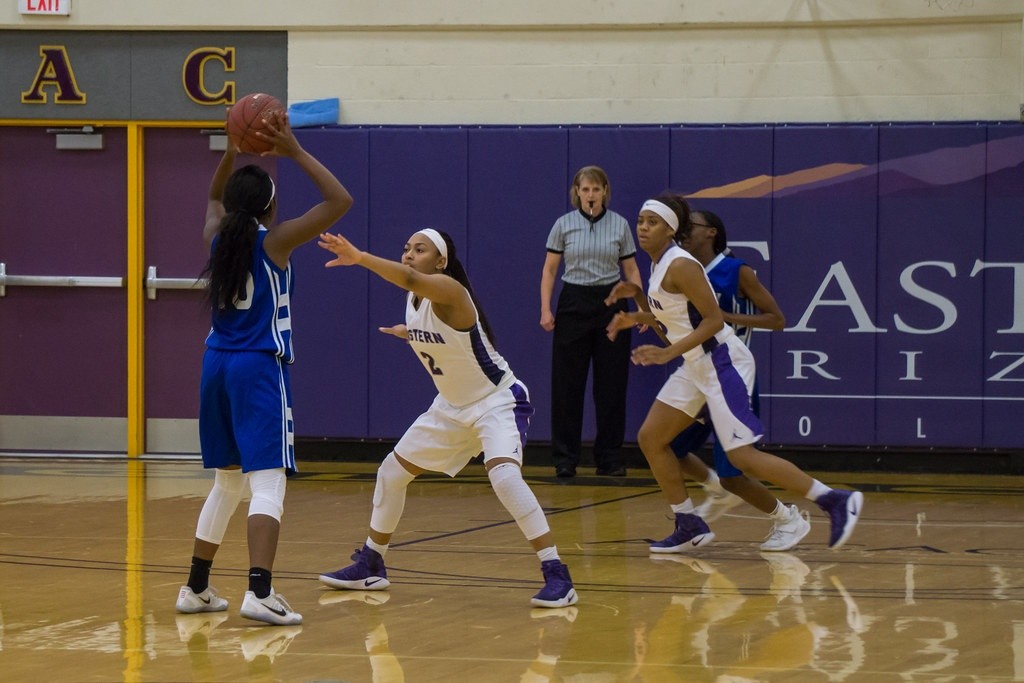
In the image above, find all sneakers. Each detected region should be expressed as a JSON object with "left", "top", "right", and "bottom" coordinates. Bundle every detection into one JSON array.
[
  {"left": 648, "top": 512, "right": 714, "bottom": 554},
  {"left": 820, "top": 561, "right": 889, "bottom": 627},
  {"left": 240, "top": 587, "right": 302, "bottom": 624},
  {"left": 761, "top": 551, "right": 811, "bottom": 585},
  {"left": 318, "top": 588, "right": 392, "bottom": 618},
  {"left": 175, "top": 610, "right": 229, "bottom": 643},
  {"left": 177, "top": 585, "right": 228, "bottom": 613},
  {"left": 650, "top": 552, "right": 712, "bottom": 596},
  {"left": 530, "top": 560, "right": 579, "bottom": 609},
  {"left": 814, "top": 489, "right": 865, "bottom": 549},
  {"left": 240, "top": 624, "right": 303, "bottom": 663},
  {"left": 531, "top": 604, "right": 577, "bottom": 656},
  {"left": 700, "top": 559, "right": 747, "bottom": 612},
  {"left": 319, "top": 546, "right": 390, "bottom": 591},
  {"left": 761, "top": 504, "right": 810, "bottom": 551},
  {"left": 695, "top": 493, "right": 742, "bottom": 522}
]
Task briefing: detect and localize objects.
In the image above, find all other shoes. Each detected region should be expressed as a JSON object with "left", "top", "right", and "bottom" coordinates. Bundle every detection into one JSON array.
[
  {"left": 557, "top": 465, "right": 576, "bottom": 478},
  {"left": 596, "top": 464, "right": 626, "bottom": 476}
]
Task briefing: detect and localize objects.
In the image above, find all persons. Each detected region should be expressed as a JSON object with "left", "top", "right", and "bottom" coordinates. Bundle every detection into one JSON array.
[
  {"left": 607, "top": 195, "right": 864, "bottom": 550},
  {"left": 176, "top": 107, "right": 354, "bottom": 624},
  {"left": 541, "top": 167, "right": 648, "bottom": 479},
  {"left": 606, "top": 210, "right": 811, "bottom": 551},
  {"left": 318, "top": 225, "right": 580, "bottom": 606}
]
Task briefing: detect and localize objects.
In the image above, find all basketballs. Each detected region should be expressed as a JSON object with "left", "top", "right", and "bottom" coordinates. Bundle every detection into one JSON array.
[{"left": 228, "top": 91, "right": 286, "bottom": 156}]
